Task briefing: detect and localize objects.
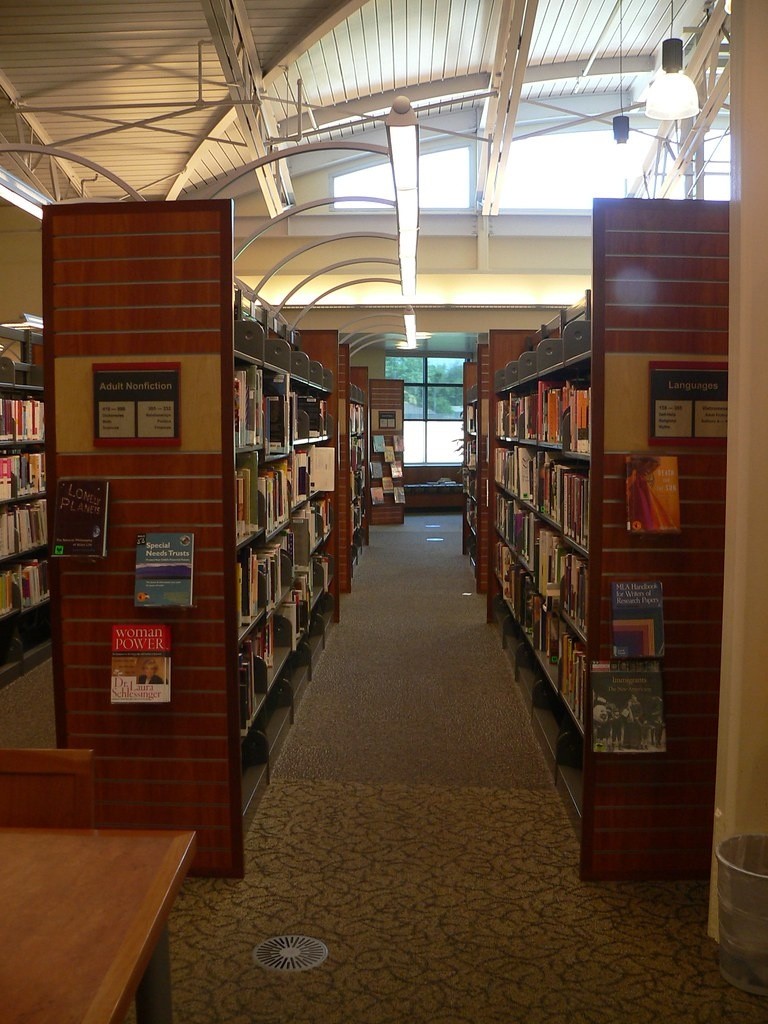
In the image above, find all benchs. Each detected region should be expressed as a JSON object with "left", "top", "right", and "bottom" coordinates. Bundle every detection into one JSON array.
[{"left": 404, "top": 466, "right": 462, "bottom": 513}]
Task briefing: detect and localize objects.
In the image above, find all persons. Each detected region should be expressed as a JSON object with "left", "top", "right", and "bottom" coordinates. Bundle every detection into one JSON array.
[{"left": 137, "top": 659, "right": 164, "bottom": 685}]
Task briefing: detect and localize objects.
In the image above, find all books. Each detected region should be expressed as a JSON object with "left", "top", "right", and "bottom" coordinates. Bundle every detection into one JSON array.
[
  {"left": 111, "top": 624, "right": 171, "bottom": 704},
  {"left": 134, "top": 533, "right": 195, "bottom": 609},
  {"left": 369, "top": 434, "right": 406, "bottom": 505},
  {"left": 50, "top": 480, "right": 108, "bottom": 558},
  {"left": 0, "top": 398, "right": 49, "bottom": 613},
  {"left": 591, "top": 660, "right": 669, "bottom": 753},
  {"left": 461, "top": 381, "right": 586, "bottom": 730},
  {"left": 227, "top": 363, "right": 370, "bottom": 729},
  {"left": 626, "top": 456, "right": 682, "bottom": 535},
  {"left": 610, "top": 578, "right": 666, "bottom": 659}
]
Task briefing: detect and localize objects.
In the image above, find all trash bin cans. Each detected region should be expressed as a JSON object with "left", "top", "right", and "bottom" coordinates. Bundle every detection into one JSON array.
[{"left": 716, "top": 833, "right": 768, "bottom": 997}]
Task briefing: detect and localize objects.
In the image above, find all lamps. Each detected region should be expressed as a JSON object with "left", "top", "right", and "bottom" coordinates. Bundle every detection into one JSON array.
[
  {"left": 206, "top": 95, "right": 420, "bottom": 330},
  {"left": 645, "top": 0, "right": 701, "bottom": 121},
  {"left": 337, "top": 305, "right": 419, "bottom": 356},
  {"left": 603, "top": 0, "right": 643, "bottom": 182}
]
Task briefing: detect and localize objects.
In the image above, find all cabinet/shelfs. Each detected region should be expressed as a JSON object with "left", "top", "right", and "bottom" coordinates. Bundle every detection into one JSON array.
[
  {"left": 0, "top": 327, "right": 51, "bottom": 692},
  {"left": 41, "top": 198, "right": 339, "bottom": 881},
  {"left": 462, "top": 344, "right": 488, "bottom": 593},
  {"left": 338, "top": 344, "right": 369, "bottom": 594},
  {"left": 370, "top": 379, "right": 405, "bottom": 525},
  {"left": 488, "top": 197, "right": 729, "bottom": 883}
]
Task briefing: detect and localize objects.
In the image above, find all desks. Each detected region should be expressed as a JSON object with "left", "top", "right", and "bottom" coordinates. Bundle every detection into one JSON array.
[{"left": 0, "top": 829, "right": 197, "bottom": 1024}]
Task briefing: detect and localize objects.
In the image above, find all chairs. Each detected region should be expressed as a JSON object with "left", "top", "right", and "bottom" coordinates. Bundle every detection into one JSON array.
[{"left": 0, "top": 749, "right": 100, "bottom": 829}]
